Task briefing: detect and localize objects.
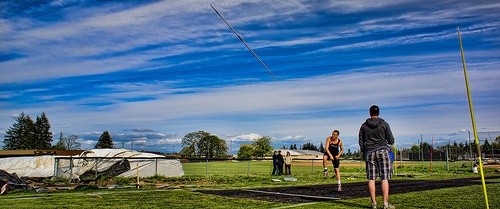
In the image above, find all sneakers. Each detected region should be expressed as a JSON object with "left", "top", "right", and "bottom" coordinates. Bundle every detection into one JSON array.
[
  {"left": 324, "top": 169, "right": 328, "bottom": 174},
  {"left": 337, "top": 184, "right": 342, "bottom": 191},
  {"left": 383, "top": 204, "right": 395, "bottom": 209},
  {"left": 371, "top": 202, "right": 377, "bottom": 209}
]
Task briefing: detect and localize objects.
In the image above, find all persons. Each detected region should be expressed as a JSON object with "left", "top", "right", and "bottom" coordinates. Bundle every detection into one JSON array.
[
  {"left": 271, "top": 150, "right": 292, "bottom": 176},
  {"left": 322, "top": 130, "right": 344, "bottom": 192},
  {"left": 359, "top": 105, "right": 395, "bottom": 209}
]
